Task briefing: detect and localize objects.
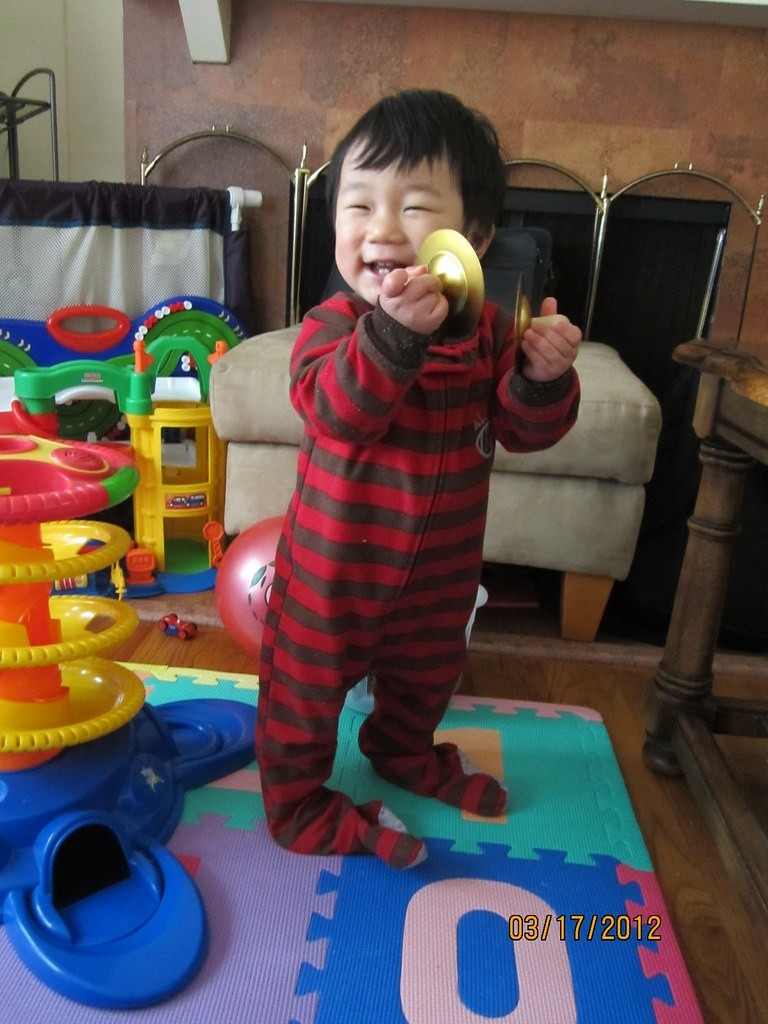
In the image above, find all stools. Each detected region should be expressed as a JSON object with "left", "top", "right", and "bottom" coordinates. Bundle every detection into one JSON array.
[{"left": 209, "top": 324, "right": 662, "bottom": 642}]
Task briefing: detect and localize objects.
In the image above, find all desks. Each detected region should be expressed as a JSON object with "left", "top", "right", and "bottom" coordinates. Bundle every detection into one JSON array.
[{"left": 641, "top": 339, "right": 768, "bottom": 967}]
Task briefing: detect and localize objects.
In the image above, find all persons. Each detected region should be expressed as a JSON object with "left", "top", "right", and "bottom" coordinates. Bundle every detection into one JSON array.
[{"left": 256, "top": 88, "right": 580, "bottom": 867}]
